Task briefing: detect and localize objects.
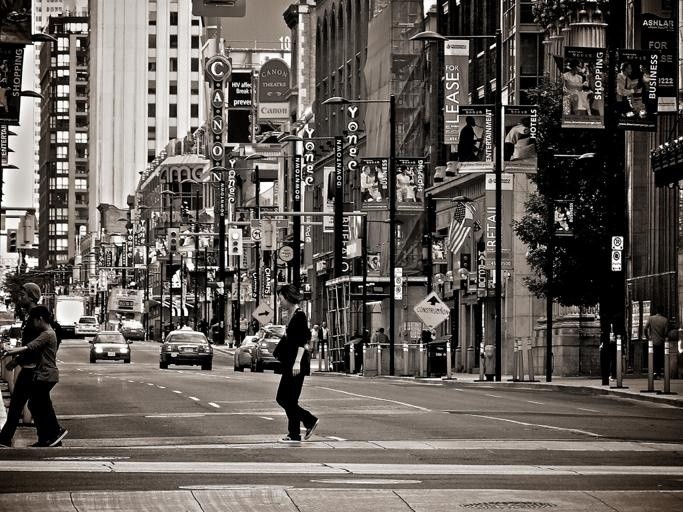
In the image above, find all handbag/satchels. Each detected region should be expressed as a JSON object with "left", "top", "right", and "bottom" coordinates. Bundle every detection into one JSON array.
[{"left": 273, "top": 335, "right": 288, "bottom": 361}]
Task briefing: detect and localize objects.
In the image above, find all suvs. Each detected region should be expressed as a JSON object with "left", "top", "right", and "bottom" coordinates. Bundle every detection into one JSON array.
[
  {"left": 73, "top": 313, "right": 101, "bottom": 341},
  {"left": 249, "top": 324, "right": 287, "bottom": 374}
]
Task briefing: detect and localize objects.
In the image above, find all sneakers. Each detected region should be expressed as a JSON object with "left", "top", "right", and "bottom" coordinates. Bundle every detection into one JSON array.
[
  {"left": 28, "top": 429, "right": 69, "bottom": 447},
  {"left": 0, "top": 432, "right": 12, "bottom": 447},
  {"left": 277, "top": 434, "right": 301, "bottom": 443},
  {"left": 304, "top": 417, "right": 320, "bottom": 439}
]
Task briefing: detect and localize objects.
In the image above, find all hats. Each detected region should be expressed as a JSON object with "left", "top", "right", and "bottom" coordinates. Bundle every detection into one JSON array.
[{"left": 23, "top": 283, "right": 41, "bottom": 301}]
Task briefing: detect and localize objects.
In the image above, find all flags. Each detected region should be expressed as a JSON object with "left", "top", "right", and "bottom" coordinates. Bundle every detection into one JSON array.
[{"left": 447, "top": 201, "right": 474, "bottom": 255}]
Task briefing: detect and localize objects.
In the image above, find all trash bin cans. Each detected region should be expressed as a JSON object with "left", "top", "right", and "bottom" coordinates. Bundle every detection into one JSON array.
[
  {"left": 426, "top": 339, "right": 448, "bottom": 378},
  {"left": 343, "top": 338, "right": 363, "bottom": 374}
]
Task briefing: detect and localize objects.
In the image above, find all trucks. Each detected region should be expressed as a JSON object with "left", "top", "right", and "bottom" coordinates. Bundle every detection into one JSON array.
[{"left": 36, "top": 293, "right": 85, "bottom": 339}]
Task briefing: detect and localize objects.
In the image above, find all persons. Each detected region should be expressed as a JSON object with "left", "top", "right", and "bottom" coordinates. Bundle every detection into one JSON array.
[
  {"left": 643, "top": 303, "right": 669, "bottom": 380},
  {"left": 0, "top": 283, "right": 63, "bottom": 448},
  {"left": 273, "top": 285, "right": 320, "bottom": 443},
  {"left": 370, "top": 257, "right": 380, "bottom": 271},
  {"left": 432, "top": 240, "right": 444, "bottom": 260},
  {"left": 395, "top": 165, "right": 418, "bottom": 202},
  {"left": 310, "top": 322, "right": 389, "bottom": 374},
  {"left": 0, "top": 307, "right": 68, "bottom": 447},
  {"left": 503, "top": 116, "right": 530, "bottom": 161},
  {"left": 561, "top": 58, "right": 594, "bottom": 117},
  {"left": 134, "top": 249, "right": 141, "bottom": 263},
  {"left": 198, "top": 227, "right": 213, "bottom": 250},
  {"left": 176, "top": 317, "right": 258, "bottom": 346},
  {"left": 360, "top": 164, "right": 388, "bottom": 202},
  {"left": 617, "top": 61, "right": 648, "bottom": 118},
  {"left": 459, "top": 116, "right": 479, "bottom": 161}
]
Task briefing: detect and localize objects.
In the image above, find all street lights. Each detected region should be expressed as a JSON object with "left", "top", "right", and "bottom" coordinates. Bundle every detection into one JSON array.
[
  {"left": 321, "top": 94, "right": 397, "bottom": 382},
  {"left": 409, "top": 28, "right": 504, "bottom": 382}
]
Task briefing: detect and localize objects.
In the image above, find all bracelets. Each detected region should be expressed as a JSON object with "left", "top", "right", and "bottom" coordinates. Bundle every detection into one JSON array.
[{"left": 295, "top": 359, "right": 300, "bottom": 364}]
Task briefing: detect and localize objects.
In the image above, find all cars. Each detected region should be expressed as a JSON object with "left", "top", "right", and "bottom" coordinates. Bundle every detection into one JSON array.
[
  {"left": 118, "top": 320, "right": 147, "bottom": 341},
  {"left": 87, "top": 330, "right": 134, "bottom": 363},
  {"left": 155, "top": 329, "right": 213, "bottom": 372},
  {"left": 232, "top": 334, "right": 259, "bottom": 373}
]
